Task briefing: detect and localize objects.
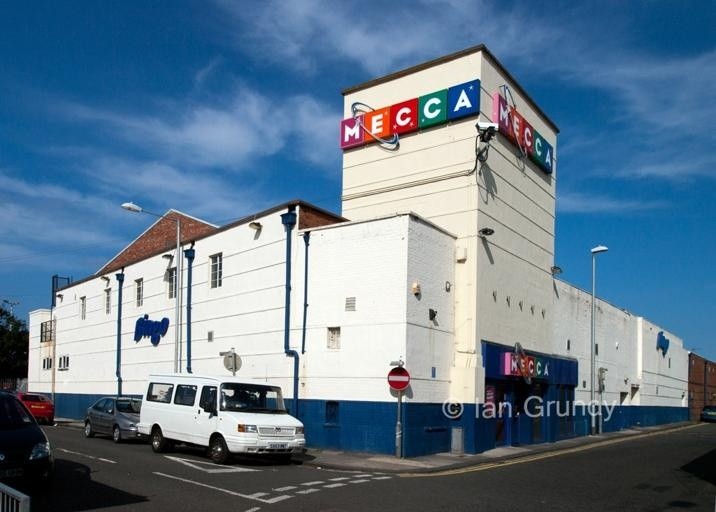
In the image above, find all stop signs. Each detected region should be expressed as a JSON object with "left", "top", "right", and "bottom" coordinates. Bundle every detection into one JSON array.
[{"left": 388, "top": 367, "right": 410, "bottom": 391}]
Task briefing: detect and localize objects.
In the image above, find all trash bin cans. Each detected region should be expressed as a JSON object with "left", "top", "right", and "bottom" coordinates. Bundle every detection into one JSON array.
[{"left": 451, "top": 426, "right": 464, "bottom": 453}]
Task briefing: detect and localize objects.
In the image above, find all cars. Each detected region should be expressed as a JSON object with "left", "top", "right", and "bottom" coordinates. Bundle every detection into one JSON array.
[
  {"left": 700, "top": 405, "right": 716, "bottom": 421},
  {"left": 84, "top": 397, "right": 151, "bottom": 444},
  {"left": 0, "top": 389, "right": 55, "bottom": 489}
]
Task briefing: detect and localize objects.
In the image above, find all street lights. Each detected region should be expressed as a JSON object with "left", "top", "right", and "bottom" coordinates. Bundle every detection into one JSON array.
[
  {"left": 2, "top": 299, "right": 20, "bottom": 331},
  {"left": 591, "top": 245, "right": 609, "bottom": 435},
  {"left": 121, "top": 202, "right": 180, "bottom": 373}
]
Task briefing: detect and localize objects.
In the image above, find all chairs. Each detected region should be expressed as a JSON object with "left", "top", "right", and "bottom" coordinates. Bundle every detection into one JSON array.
[{"left": 168, "top": 386, "right": 258, "bottom": 413}]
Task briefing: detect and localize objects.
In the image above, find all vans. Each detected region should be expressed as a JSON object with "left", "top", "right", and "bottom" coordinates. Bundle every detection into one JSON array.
[{"left": 137, "top": 373, "right": 307, "bottom": 464}]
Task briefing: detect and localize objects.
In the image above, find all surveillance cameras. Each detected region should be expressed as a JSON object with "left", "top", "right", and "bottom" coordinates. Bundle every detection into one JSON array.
[{"left": 475, "top": 122, "right": 499, "bottom": 141}]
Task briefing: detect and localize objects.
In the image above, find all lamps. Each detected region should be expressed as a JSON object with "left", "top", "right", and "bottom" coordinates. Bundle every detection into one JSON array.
[
  {"left": 249, "top": 222, "right": 261, "bottom": 229},
  {"left": 162, "top": 254, "right": 173, "bottom": 259},
  {"left": 101, "top": 277, "right": 109, "bottom": 281},
  {"left": 551, "top": 266, "right": 562, "bottom": 274},
  {"left": 479, "top": 227, "right": 494, "bottom": 236},
  {"left": 56, "top": 294, "right": 63, "bottom": 297}
]
